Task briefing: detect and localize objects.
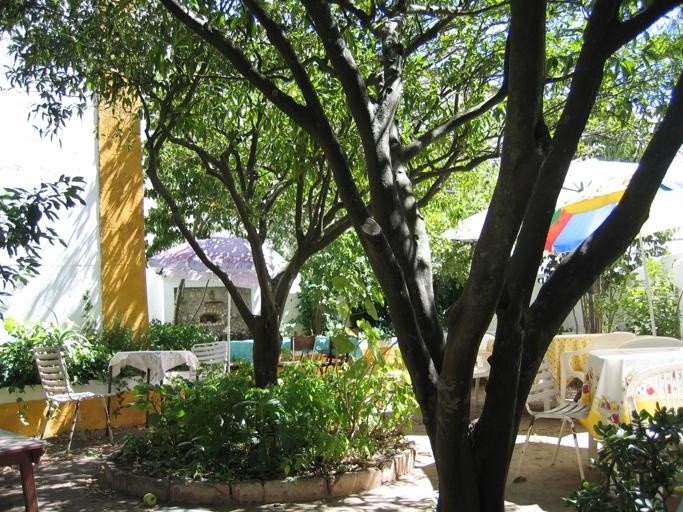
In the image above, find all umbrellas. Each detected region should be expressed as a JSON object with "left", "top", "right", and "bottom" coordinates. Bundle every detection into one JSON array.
[
  {"left": 544, "top": 181, "right": 683, "bottom": 336},
  {"left": 149, "top": 237, "right": 301, "bottom": 373},
  {"left": 441, "top": 158, "right": 639, "bottom": 240}
]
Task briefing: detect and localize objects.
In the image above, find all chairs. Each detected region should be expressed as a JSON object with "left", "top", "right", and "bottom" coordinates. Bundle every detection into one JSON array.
[
  {"left": 187, "top": 341, "right": 228, "bottom": 383},
  {"left": 33, "top": 345, "right": 114, "bottom": 451},
  {"left": 470, "top": 332, "right": 496, "bottom": 414},
  {"left": 231, "top": 335, "right": 399, "bottom": 380}
]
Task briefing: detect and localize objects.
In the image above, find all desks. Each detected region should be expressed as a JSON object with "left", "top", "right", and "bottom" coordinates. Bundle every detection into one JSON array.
[
  {"left": 106, "top": 350, "right": 192, "bottom": 436},
  {"left": 0, "top": 429, "right": 47, "bottom": 512},
  {"left": 517, "top": 331, "right": 682, "bottom": 485}
]
